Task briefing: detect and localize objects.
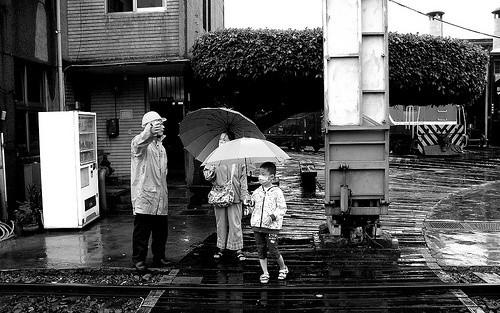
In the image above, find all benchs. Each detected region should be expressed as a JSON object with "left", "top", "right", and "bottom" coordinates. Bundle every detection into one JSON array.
[
  {"left": 187, "top": 182, "right": 279, "bottom": 208},
  {"left": 298, "top": 159, "right": 317, "bottom": 193}
]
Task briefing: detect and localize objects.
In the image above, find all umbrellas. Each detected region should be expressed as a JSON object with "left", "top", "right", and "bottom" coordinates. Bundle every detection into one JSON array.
[
  {"left": 199, "top": 135, "right": 291, "bottom": 203},
  {"left": 177, "top": 105, "right": 267, "bottom": 169}
]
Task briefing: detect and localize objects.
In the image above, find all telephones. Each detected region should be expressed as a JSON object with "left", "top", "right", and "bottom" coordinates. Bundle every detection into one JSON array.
[{"left": 105, "top": 118, "right": 120, "bottom": 139}]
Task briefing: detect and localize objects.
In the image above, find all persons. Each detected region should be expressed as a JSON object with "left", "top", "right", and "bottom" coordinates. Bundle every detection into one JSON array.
[
  {"left": 244, "top": 162, "right": 289, "bottom": 284},
  {"left": 130, "top": 111, "right": 173, "bottom": 271},
  {"left": 203, "top": 130, "right": 249, "bottom": 262}
]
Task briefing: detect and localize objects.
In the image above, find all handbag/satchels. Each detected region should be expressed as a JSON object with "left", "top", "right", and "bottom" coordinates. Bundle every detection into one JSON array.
[{"left": 207, "top": 183, "right": 235, "bottom": 208}]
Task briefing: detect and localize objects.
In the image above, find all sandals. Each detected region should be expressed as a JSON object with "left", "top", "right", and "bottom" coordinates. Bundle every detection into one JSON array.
[
  {"left": 259, "top": 272, "right": 270, "bottom": 283},
  {"left": 213, "top": 251, "right": 224, "bottom": 259},
  {"left": 235, "top": 249, "right": 246, "bottom": 262},
  {"left": 278, "top": 266, "right": 289, "bottom": 281}
]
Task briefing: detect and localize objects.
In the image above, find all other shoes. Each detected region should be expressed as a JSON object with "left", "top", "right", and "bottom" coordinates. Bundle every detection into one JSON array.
[
  {"left": 135, "top": 262, "right": 147, "bottom": 272},
  {"left": 154, "top": 258, "right": 171, "bottom": 265}
]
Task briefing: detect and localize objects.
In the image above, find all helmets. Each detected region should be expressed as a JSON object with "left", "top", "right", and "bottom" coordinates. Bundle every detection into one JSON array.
[{"left": 141, "top": 111, "right": 167, "bottom": 127}]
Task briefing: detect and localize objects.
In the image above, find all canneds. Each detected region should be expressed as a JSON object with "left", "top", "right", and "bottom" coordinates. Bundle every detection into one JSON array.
[{"left": 80, "top": 141, "right": 91, "bottom": 162}]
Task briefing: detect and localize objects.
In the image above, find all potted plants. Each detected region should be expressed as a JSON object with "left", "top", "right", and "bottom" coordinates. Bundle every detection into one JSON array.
[{"left": 14, "top": 200, "right": 40, "bottom": 232}]
